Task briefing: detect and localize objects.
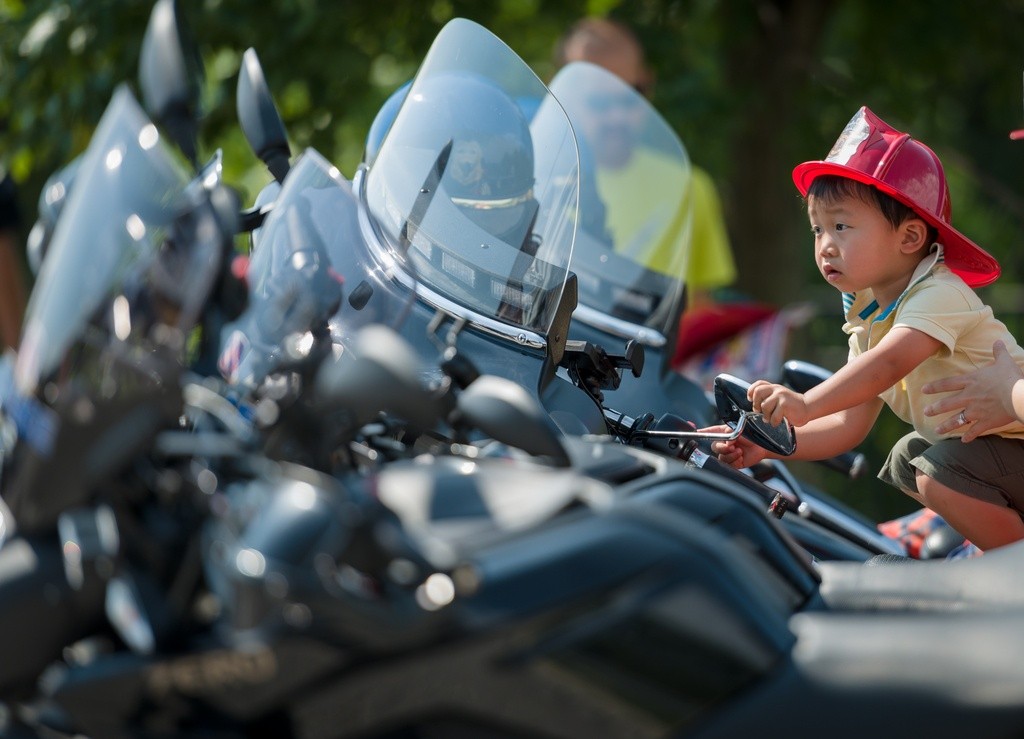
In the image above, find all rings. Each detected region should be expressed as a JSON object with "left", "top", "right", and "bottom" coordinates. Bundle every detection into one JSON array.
[{"left": 960, "top": 410, "right": 971, "bottom": 424}]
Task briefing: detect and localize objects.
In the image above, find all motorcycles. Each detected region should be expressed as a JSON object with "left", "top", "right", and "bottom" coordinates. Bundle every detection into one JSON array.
[{"left": 1, "top": 1, "right": 1023, "bottom": 738}]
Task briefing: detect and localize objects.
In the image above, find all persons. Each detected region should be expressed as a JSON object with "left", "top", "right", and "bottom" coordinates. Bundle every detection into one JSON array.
[
  {"left": 698, "top": 104, "right": 1024, "bottom": 549},
  {"left": 921, "top": 340, "right": 1024, "bottom": 442},
  {"left": 562, "top": 12, "right": 737, "bottom": 312},
  {"left": 0, "top": 177, "right": 56, "bottom": 450}
]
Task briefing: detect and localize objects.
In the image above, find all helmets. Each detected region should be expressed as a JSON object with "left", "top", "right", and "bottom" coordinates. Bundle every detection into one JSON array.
[
  {"left": 367, "top": 77, "right": 535, "bottom": 236},
  {"left": 791, "top": 106, "right": 1001, "bottom": 288}
]
